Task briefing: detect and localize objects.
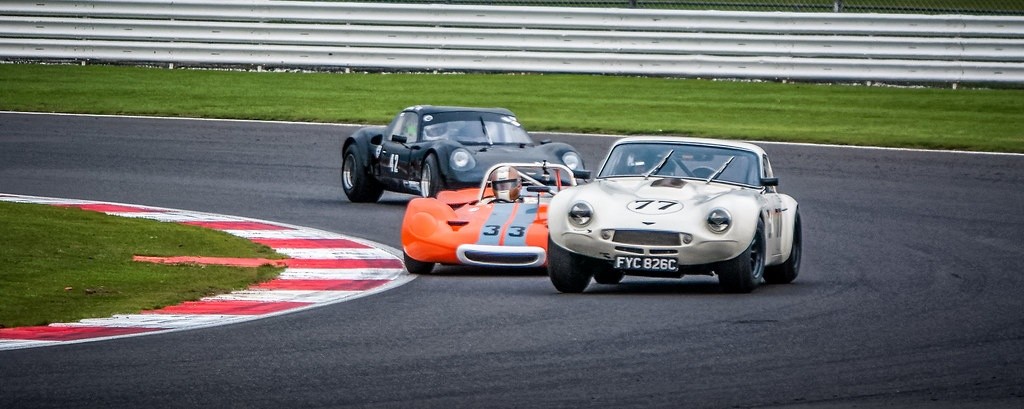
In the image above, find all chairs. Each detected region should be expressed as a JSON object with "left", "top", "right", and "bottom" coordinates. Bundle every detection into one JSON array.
[{"left": 644, "top": 157, "right": 676, "bottom": 176}]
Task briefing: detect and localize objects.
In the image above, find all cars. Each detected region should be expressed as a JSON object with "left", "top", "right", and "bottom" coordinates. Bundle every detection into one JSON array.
[
  {"left": 547, "top": 134, "right": 802, "bottom": 293},
  {"left": 342, "top": 105, "right": 586, "bottom": 204},
  {"left": 402, "top": 162, "right": 581, "bottom": 275}
]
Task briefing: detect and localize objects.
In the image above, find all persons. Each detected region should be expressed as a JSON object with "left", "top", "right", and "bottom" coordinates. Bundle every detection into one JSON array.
[
  {"left": 477, "top": 166, "right": 524, "bottom": 205},
  {"left": 423, "top": 122, "right": 449, "bottom": 140}
]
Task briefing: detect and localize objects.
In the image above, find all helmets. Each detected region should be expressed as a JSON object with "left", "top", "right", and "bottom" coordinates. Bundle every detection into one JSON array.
[
  {"left": 627, "top": 152, "right": 648, "bottom": 174},
  {"left": 423, "top": 122, "right": 447, "bottom": 140},
  {"left": 490, "top": 166, "right": 522, "bottom": 201}
]
